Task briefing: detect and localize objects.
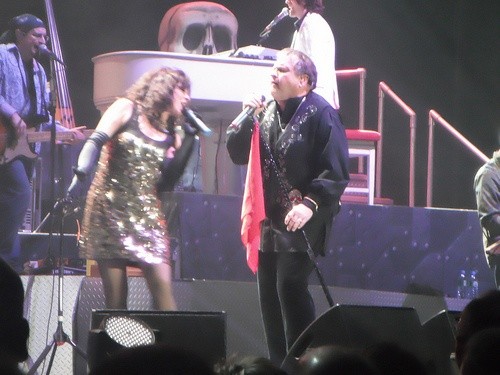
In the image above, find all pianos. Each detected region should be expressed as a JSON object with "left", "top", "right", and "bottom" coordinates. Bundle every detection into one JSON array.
[{"left": 90, "top": 43, "right": 300, "bottom": 193}]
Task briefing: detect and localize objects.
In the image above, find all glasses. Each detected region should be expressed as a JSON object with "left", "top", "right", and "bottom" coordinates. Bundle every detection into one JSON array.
[{"left": 17, "top": 29, "right": 49, "bottom": 42}]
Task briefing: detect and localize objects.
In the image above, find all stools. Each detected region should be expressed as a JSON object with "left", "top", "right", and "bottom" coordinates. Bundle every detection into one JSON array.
[{"left": 341, "top": 128, "right": 380, "bottom": 206}]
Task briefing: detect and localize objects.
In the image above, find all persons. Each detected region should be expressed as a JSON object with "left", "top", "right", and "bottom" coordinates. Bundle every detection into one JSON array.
[
  {"left": 213, "top": 354, "right": 289, "bottom": 375},
  {"left": 296, "top": 344, "right": 380, "bottom": 375},
  {"left": 225, "top": 49, "right": 351, "bottom": 368},
  {"left": 0, "top": 257, "right": 30, "bottom": 375},
  {"left": 474, "top": 150, "right": 500, "bottom": 288},
  {"left": 455, "top": 290, "right": 500, "bottom": 375},
  {"left": 0, "top": 14, "right": 87, "bottom": 275},
  {"left": 286, "top": 0, "right": 340, "bottom": 109},
  {"left": 64, "top": 65, "right": 203, "bottom": 310},
  {"left": 361, "top": 341, "right": 426, "bottom": 375}
]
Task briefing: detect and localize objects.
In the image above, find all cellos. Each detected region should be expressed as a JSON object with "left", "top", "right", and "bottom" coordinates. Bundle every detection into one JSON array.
[{"left": 41, "top": 0, "right": 88, "bottom": 183}]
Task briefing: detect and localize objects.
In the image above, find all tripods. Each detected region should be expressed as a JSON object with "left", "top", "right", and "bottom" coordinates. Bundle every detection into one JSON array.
[{"left": 26, "top": 142, "right": 89, "bottom": 375}]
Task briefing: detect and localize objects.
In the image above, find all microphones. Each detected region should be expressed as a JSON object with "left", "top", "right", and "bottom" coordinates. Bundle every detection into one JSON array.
[
  {"left": 39, "top": 44, "right": 66, "bottom": 67},
  {"left": 183, "top": 105, "right": 212, "bottom": 136},
  {"left": 228, "top": 95, "right": 265, "bottom": 130},
  {"left": 260, "top": 7, "right": 291, "bottom": 38}
]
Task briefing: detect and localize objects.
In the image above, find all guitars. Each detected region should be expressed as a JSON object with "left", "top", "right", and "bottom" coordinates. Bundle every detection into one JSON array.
[{"left": 0, "top": 115, "right": 101, "bottom": 168}]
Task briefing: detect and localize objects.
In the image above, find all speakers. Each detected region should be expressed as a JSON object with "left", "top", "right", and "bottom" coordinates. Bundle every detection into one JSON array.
[
  {"left": 420, "top": 309, "right": 461, "bottom": 375},
  {"left": 88, "top": 309, "right": 227, "bottom": 374},
  {"left": 280, "top": 302, "right": 436, "bottom": 375}
]
